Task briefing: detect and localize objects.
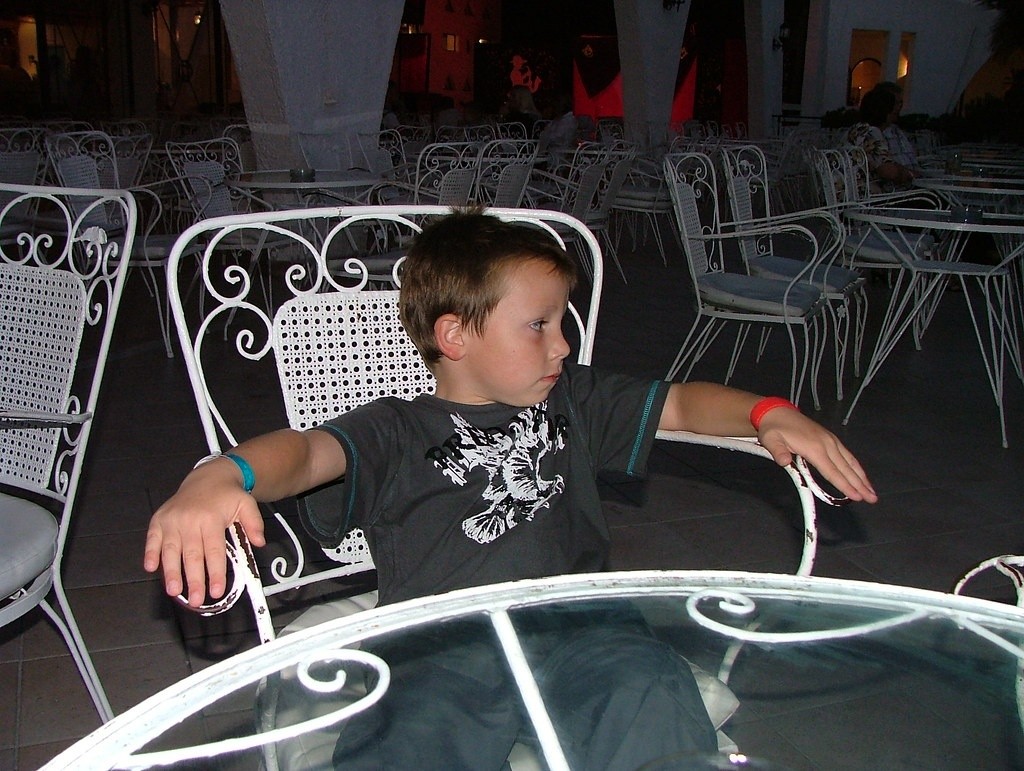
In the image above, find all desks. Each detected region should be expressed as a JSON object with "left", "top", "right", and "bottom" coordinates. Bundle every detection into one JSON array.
[
  {"left": 844, "top": 142, "right": 1024, "bottom": 449},
  {"left": 34, "top": 570, "right": 1024, "bottom": 770},
  {"left": 222, "top": 168, "right": 388, "bottom": 341},
  {"left": 408, "top": 151, "right": 551, "bottom": 207},
  {"left": 554, "top": 146, "right": 641, "bottom": 155},
  {"left": 772, "top": 114, "right": 823, "bottom": 148}
]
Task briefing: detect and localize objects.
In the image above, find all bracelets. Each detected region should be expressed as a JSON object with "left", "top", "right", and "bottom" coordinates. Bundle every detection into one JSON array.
[
  {"left": 191, "top": 451, "right": 256, "bottom": 494},
  {"left": 751, "top": 396, "right": 796, "bottom": 427}
]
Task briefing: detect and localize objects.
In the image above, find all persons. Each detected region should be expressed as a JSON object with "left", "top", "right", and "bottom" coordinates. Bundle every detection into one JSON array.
[{"left": 144, "top": 205, "right": 879, "bottom": 771}]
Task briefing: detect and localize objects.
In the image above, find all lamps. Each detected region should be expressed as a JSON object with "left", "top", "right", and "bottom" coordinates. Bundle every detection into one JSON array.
[{"left": 772, "top": 22, "right": 791, "bottom": 51}]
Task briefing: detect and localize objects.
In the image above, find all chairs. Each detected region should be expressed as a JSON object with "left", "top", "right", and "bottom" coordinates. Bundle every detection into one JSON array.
[
  {"left": 1, "top": 180, "right": 138, "bottom": 725},
  {"left": 166, "top": 204, "right": 858, "bottom": 770},
  {"left": 1, "top": 114, "right": 939, "bottom": 411}
]
